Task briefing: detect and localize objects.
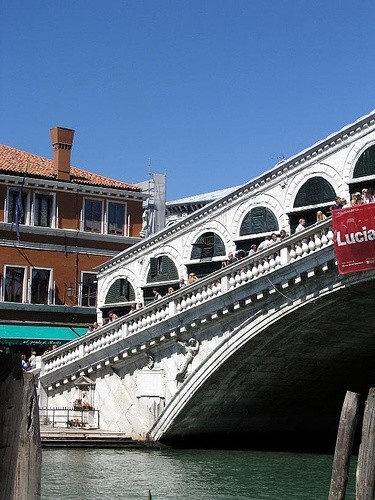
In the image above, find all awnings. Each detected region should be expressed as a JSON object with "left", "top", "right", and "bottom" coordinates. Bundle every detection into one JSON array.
[{"left": 0, "top": 324, "right": 89, "bottom": 345}]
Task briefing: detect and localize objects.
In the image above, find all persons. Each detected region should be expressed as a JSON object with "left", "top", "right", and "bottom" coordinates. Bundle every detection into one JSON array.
[
  {"left": 153, "top": 288, "right": 162, "bottom": 301},
  {"left": 166, "top": 287, "right": 174, "bottom": 295},
  {"left": 351, "top": 188, "right": 375, "bottom": 206},
  {"left": 257, "top": 229, "right": 289, "bottom": 252},
  {"left": 179, "top": 278, "right": 186, "bottom": 288},
  {"left": 22, "top": 351, "right": 36, "bottom": 371},
  {"left": 189, "top": 273, "right": 198, "bottom": 285},
  {"left": 177, "top": 338, "right": 199, "bottom": 375},
  {"left": 129, "top": 303, "right": 142, "bottom": 313},
  {"left": 317, "top": 211, "right": 326, "bottom": 222},
  {"left": 329, "top": 196, "right": 351, "bottom": 210},
  {"left": 86, "top": 322, "right": 101, "bottom": 334},
  {"left": 222, "top": 244, "right": 256, "bottom": 266},
  {"left": 103, "top": 311, "right": 118, "bottom": 325},
  {"left": 295, "top": 218, "right": 305, "bottom": 233}
]
